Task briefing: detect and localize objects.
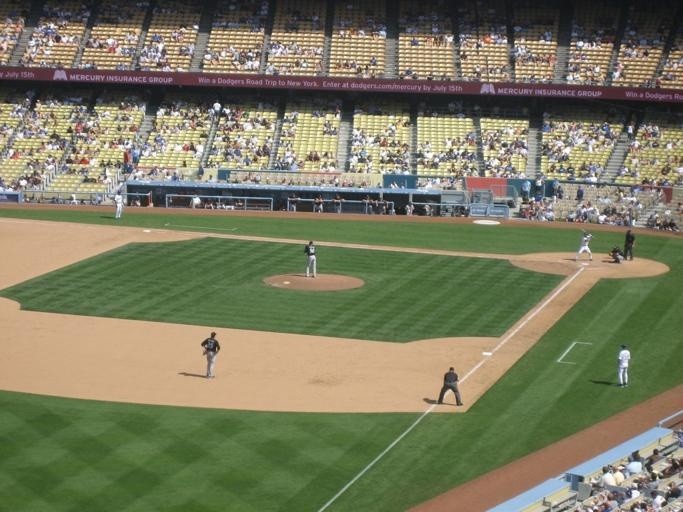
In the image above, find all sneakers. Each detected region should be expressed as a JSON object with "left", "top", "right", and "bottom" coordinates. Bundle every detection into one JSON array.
[
  {"left": 114, "top": 216, "right": 120, "bottom": 220},
  {"left": 573, "top": 257, "right": 633, "bottom": 264},
  {"left": 437, "top": 400, "right": 442, "bottom": 404},
  {"left": 616, "top": 383, "right": 628, "bottom": 388},
  {"left": 456, "top": 402, "right": 463, "bottom": 406},
  {"left": 206, "top": 374, "right": 215, "bottom": 378},
  {"left": 305, "top": 275, "right": 316, "bottom": 279}
]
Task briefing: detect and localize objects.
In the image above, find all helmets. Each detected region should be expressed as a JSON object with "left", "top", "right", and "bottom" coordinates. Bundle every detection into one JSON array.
[{"left": 583, "top": 232, "right": 588, "bottom": 237}]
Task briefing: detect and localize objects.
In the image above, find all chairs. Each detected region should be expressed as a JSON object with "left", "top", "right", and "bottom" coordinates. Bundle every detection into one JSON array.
[
  {"left": 1, "top": 1, "right": 330, "bottom": 205},
  {"left": 327, "top": 2, "right": 682, "bottom": 230}
]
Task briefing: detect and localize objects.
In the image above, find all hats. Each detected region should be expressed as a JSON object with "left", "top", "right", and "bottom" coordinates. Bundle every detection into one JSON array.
[
  {"left": 449, "top": 367, "right": 454, "bottom": 370},
  {"left": 211, "top": 332, "right": 216, "bottom": 336}
]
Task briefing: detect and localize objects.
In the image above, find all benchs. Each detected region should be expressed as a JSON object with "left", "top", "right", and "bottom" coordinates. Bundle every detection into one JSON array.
[{"left": 540, "top": 433, "right": 683, "bottom": 511}]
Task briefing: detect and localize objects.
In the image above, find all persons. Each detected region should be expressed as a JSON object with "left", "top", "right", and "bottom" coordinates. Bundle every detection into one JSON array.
[
  {"left": 618, "top": 346, "right": 631, "bottom": 388},
  {"left": 573, "top": 232, "right": 592, "bottom": 261},
  {"left": 609, "top": 245, "right": 625, "bottom": 264},
  {"left": 574, "top": 449, "right": 683, "bottom": 511},
  {"left": 623, "top": 230, "right": 635, "bottom": 261},
  {"left": 303, "top": 241, "right": 317, "bottom": 278},
  {"left": 201, "top": 332, "right": 220, "bottom": 378},
  {"left": 437, "top": 367, "right": 463, "bottom": 406}
]
